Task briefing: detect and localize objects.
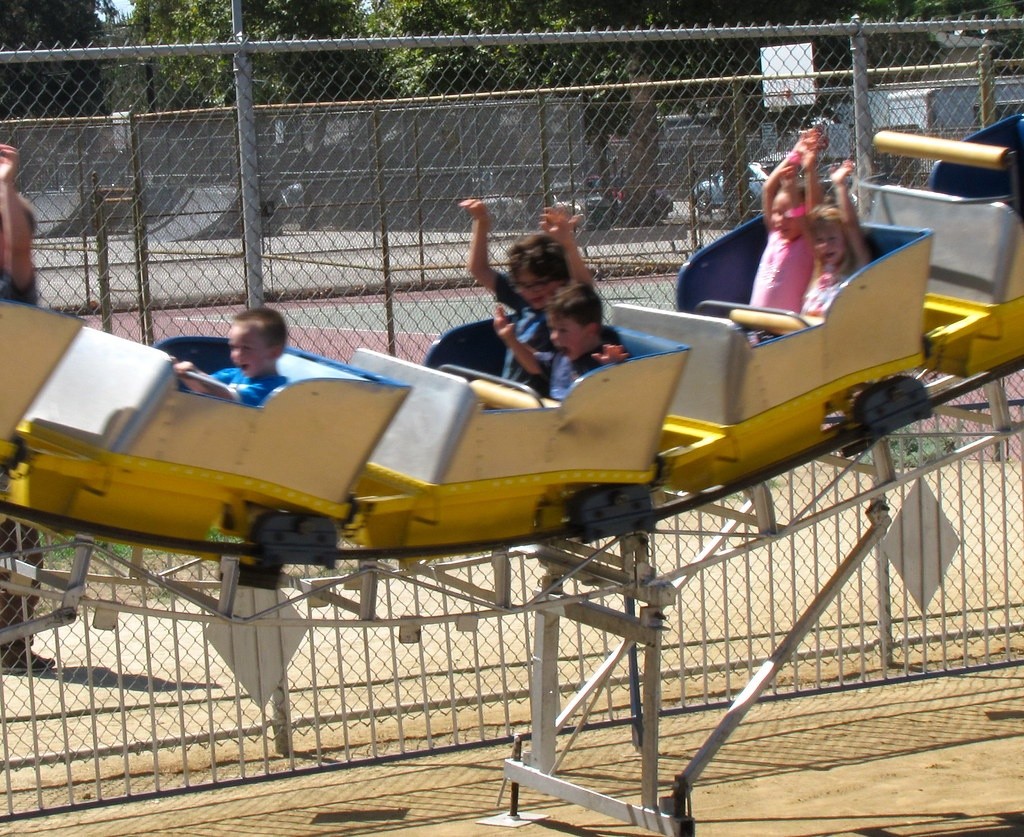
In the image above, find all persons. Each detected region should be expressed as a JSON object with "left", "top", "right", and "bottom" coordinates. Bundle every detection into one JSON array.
[
  {"left": 0, "top": 143, "right": 39, "bottom": 304},
  {"left": 0, "top": 518, "right": 55, "bottom": 674},
  {"left": 457, "top": 199, "right": 593, "bottom": 396},
  {"left": 749, "top": 129, "right": 830, "bottom": 314},
  {"left": 800, "top": 148, "right": 870, "bottom": 319},
  {"left": 169, "top": 308, "right": 287, "bottom": 406},
  {"left": 494, "top": 282, "right": 628, "bottom": 402}
]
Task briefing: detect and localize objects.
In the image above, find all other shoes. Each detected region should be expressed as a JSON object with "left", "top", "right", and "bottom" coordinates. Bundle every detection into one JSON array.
[{"left": 1, "top": 649, "right": 55, "bottom": 673}]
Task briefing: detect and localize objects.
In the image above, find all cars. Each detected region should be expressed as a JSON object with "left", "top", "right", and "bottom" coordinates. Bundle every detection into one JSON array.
[
  {"left": 817, "top": 163, "right": 887, "bottom": 203},
  {"left": 690, "top": 160, "right": 777, "bottom": 216},
  {"left": 579, "top": 176, "right": 674, "bottom": 220}
]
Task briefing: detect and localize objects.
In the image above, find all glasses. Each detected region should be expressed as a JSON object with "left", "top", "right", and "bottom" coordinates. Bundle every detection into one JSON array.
[{"left": 510, "top": 277, "right": 554, "bottom": 293}]
